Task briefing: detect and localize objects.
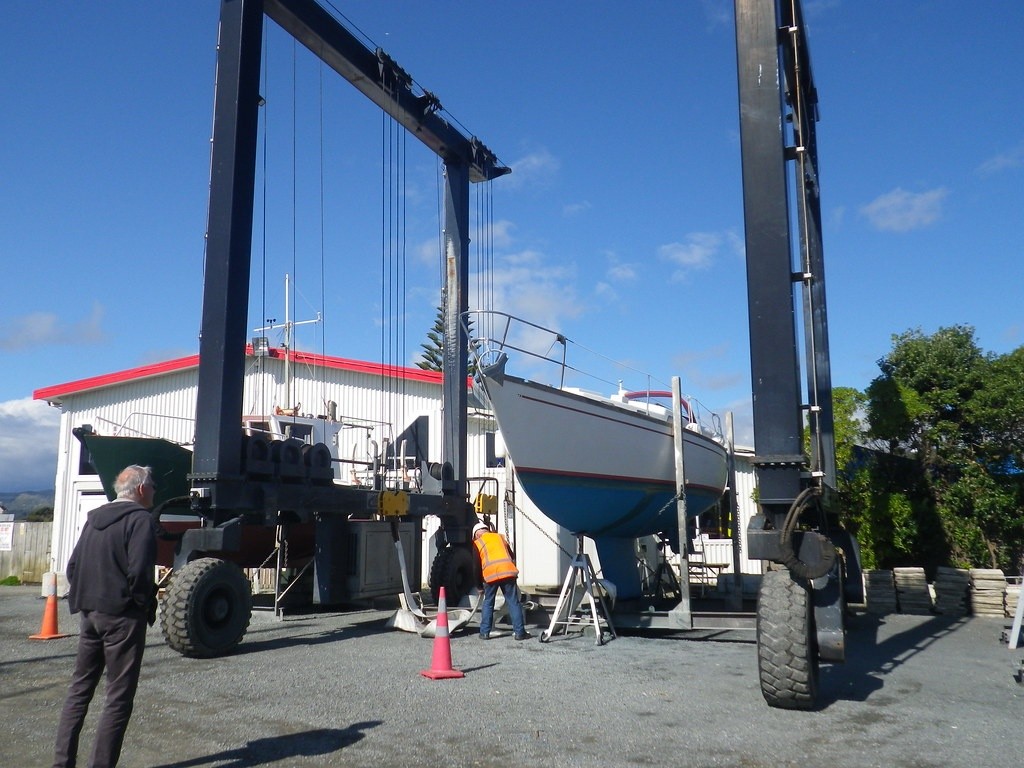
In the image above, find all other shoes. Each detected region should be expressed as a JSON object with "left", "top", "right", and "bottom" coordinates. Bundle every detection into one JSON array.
[
  {"left": 480, "top": 634, "right": 491, "bottom": 640},
  {"left": 515, "top": 633, "right": 531, "bottom": 640}
]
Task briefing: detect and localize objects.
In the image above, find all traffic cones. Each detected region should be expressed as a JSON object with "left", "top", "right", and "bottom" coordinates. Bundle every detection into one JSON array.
[
  {"left": 27, "top": 571, "right": 74, "bottom": 641},
  {"left": 423, "top": 586, "right": 465, "bottom": 680}
]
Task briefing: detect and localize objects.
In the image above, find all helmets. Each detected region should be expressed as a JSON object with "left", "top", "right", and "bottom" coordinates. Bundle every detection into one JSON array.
[{"left": 471, "top": 523, "right": 489, "bottom": 540}]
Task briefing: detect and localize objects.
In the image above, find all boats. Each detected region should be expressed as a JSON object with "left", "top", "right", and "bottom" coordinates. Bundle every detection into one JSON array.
[{"left": 459, "top": 306, "right": 734, "bottom": 534}]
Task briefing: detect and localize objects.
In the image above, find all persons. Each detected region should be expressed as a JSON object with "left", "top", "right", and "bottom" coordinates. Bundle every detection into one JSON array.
[
  {"left": 472, "top": 522, "right": 532, "bottom": 640},
  {"left": 52, "top": 466, "right": 162, "bottom": 768}
]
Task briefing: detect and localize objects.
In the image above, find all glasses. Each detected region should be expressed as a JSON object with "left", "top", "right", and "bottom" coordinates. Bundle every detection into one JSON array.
[{"left": 145, "top": 482, "right": 157, "bottom": 490}]
